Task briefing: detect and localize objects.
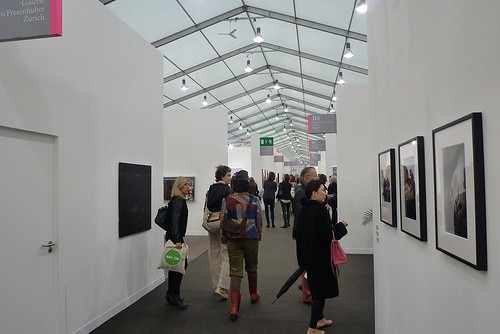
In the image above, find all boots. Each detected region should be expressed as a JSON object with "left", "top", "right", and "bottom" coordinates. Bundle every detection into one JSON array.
[{"left": 166, "top": 262, "right": 188, "bottom": 308}]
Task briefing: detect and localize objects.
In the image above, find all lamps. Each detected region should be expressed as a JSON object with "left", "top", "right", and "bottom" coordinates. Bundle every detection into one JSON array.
[{"left": 180, "top": 0, "right": 370, "bottom": 163}]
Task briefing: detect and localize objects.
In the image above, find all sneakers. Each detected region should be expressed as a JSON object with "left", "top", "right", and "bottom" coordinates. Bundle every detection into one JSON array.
[{"left": 215, "top": 288, "right": 228, "bottom": 299}]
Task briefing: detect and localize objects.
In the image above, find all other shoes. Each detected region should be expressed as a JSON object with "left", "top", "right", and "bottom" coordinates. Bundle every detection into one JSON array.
[
  {"left": 287, "top": 224, "right": 290, "bottom": 226},
  {"left": 298, "top": 285, "right": 302, "bottom": 290},
  {"left": 280, "top": 226, "right": 287, "bottom": 228},
  {"left": 266, "top": 225, "right": 270, "bottom": 227},
  {"left": 229, "top": 306, "right": 239, "bottom": 320},
  {"left": 251, "top": 291, "right": 260, "bottom": 301},
  {"left": 303, "top": 300, "right": 313, "bottom": 305},
  {"left": 307, "top": 328, "right": 325, "bottom": 334},
  {"left": 272, "top": 225, "right": 275, "bottom": 227},
  {"left": 317, "top": 320, "right": 332, "bottom": 327}
]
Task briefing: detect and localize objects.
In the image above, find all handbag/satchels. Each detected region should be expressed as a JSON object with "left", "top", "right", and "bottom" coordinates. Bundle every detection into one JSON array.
[
  {"left": 202, "top": 196, "right": 222, "bottom": 233},
  {"left": 154, "top": 202, "right": 171, "bottom": 231},
  {"left": 157, "top": 239, "right": 189, "bottom": 274},
  {"left": 331, "top": 230, "right": 347, "bottom": 265}
]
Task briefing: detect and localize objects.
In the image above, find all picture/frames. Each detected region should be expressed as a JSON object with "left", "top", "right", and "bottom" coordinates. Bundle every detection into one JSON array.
[
  {"left": 378, "top": 149, "right": 397, "bottom": 227},
  {"left": 431, "top": 112, "right": 487, "bottom": 272},
  {"left": 398, "top": 136, "right": 427, "bottom": 241}
]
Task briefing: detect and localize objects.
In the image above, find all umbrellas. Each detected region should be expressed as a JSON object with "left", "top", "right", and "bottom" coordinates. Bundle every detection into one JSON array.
[{"left": 271, "top": 266, "right": 306, "bottom": 306}]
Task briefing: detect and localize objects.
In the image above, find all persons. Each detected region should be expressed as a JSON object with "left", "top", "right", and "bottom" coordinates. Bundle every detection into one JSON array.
[
  {"left": 292, "top": 179, "right": 348, "bottom": 334},
  {"left": 292, "top": 166, "right": 336, "bottom": 304},
  {"left": 155, "top": 176, "right": 190, "bottom": 309},
  {"left": 263, "top": 172, "right": 338, "bottom": 229},
  {"left": 219, "top": 169, "right": 264, "bottom": 320},
  {"left": 402, "top": 165, "right": 416, "bottom": 220},
  {"left": 202, "top": 165, "right": 231, "bottom": 300}
]
